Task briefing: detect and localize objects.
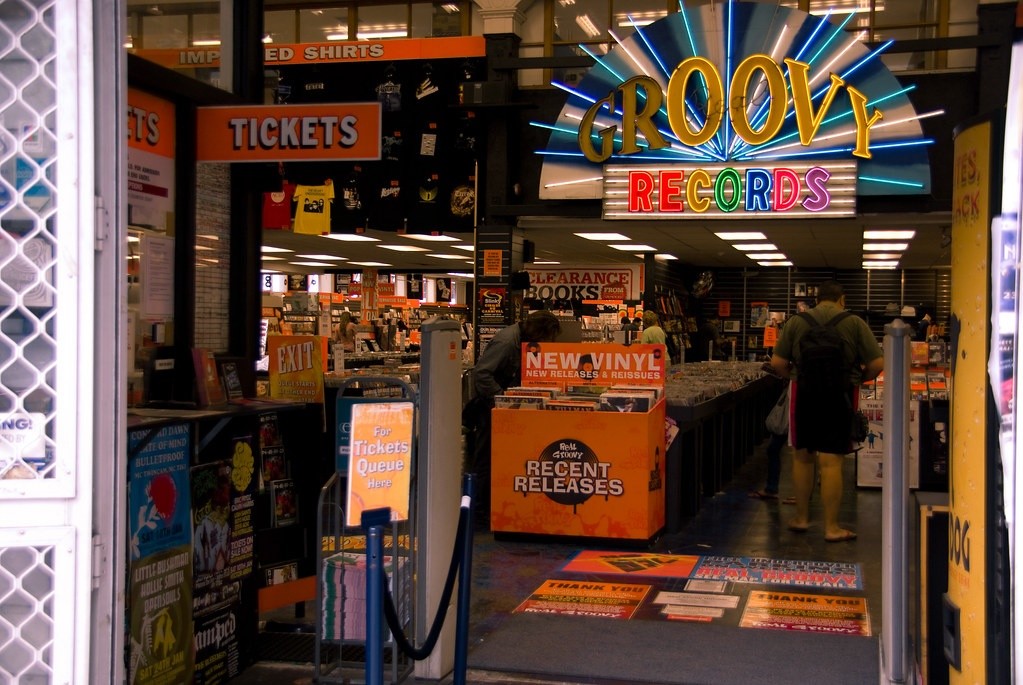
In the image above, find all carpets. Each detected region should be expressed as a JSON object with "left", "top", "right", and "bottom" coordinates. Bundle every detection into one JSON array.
[{"left": 467, "top": 611, "right": 880, "bottom": 685}]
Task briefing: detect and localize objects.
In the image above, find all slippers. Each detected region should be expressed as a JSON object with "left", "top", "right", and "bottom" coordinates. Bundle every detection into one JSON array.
[
  {"left": 748, "top": 490, "right": 780, "bottom": 500},
  {"left": 824, "top": 528, "right": 857, "bottom": 541},
  {"left": 787, "top": 516, "right": 810, "bottom": 532}
]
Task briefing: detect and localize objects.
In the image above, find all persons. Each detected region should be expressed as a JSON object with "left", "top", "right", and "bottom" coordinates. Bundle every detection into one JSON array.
[
  {"left": 620, "top": 310, "right": 671, "bottom": 375},
  {"left": 378, "top": 313, "right": 393, "bottom": 339},
  {"left": 746, "top": 281, "right": 885, "bottom": 542},
  {"left": 397, "top": 320, "right": 410, "bottom": 337},
  {"left": 334, "top": 311, "right": 359, "bottom": 354},
  {"left": 698, "top": 313, "right": 728, "bottom": 362},
  {"left": 602, "top": 397, "right": 637, "bottom": 412},
  {"left": 467, "top": 310, "right": 562, "bottom": 530}
]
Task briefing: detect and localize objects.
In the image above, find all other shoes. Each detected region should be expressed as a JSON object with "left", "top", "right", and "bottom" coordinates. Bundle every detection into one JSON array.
[{"left": 783, "top": 494, "right": 797, "bottom": 504}]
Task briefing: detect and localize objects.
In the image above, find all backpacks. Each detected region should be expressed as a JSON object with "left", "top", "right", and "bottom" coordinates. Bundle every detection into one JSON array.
[{"left": 793, "top": 311, "right": 851, "bottom": 393}]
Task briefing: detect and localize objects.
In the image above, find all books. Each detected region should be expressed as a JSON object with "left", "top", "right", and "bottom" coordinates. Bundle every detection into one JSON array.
[
  {"left": 858, "top": 340, "right": 951, "bottom": 400},
  {"left": 493, "top": 383, "right": 663, "bottom": 413}
]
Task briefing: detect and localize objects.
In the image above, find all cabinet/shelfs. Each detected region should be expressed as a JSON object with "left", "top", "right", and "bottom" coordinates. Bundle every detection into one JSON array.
[
  {"left": 314, "top": 470, "right": 417, "bottom": 684},
  {"left": 855, "top": 341, "right": 952, "bottom": 491},
  {"left": 907, "top": 492, "right": 949, "bottom": 683},
  {"left": 260, "top": 282, "right": 703, "bottom": 481}
]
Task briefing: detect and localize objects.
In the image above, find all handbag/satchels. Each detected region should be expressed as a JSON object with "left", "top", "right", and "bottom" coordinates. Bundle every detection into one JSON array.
[
  {"left": 765, "top": 387, "right": 789, "bottom": 435},
  {"left": 462, "top": 367, "right": 490, "bottom": 430},
  {"left": 849, "top": 411, "right": 870, "bottom": 442}
]
